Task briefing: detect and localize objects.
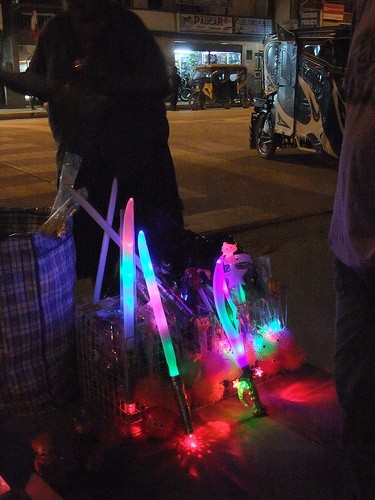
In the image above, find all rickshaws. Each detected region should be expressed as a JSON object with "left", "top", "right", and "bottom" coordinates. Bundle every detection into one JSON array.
[{"left": 179, "top": 73, "right": 193, "bottom": 102}]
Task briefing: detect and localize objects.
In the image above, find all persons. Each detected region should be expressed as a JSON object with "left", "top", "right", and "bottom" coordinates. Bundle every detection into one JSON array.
[
  {"left": 324, "top": 0, "right": 375, "bottom": 500},
  {"left": 25, "top": 65, "right": 37, "bottom": 112},
  {"left": 0, "top": 0, "right": 184, "bottom": 303}
]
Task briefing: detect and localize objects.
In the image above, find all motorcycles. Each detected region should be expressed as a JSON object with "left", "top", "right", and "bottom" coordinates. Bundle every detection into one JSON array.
[
  {"left": 247, "top": 22, "right": 354, "bottom": 162},
  {"left": 188, "top": 63, "right": 252, "bottom": 111}
]
